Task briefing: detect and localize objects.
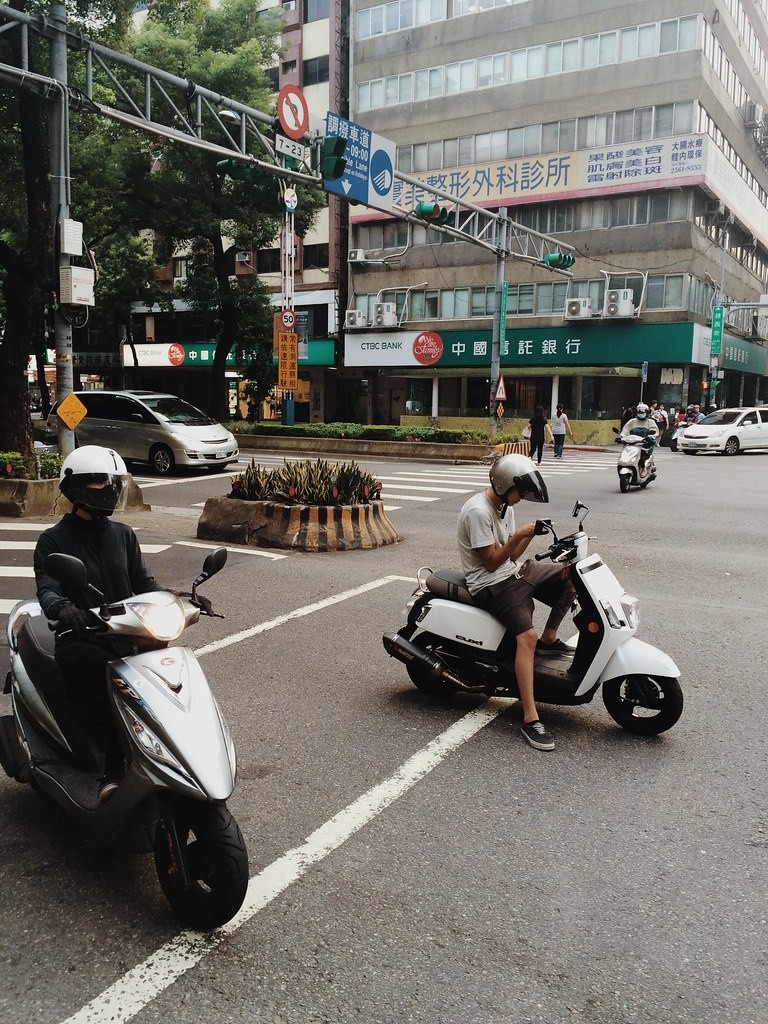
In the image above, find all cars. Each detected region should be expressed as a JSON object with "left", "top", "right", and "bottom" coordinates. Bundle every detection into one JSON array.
[{"left": 676, "top": 407, "right": 768, "bottom": 456}]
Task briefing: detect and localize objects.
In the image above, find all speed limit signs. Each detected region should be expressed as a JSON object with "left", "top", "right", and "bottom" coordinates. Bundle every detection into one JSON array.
[{"left": 279, "top": 309, "right": 297, "bottom": 331}]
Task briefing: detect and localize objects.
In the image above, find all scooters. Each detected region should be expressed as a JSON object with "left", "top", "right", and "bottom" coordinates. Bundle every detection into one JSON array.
[
  {"left": 29, "top": 393, "right": 44, "bottom": 413},
  {"left": 670, "top": 417, "right": 700, "bottom": 452},
  {"left": 382, "top": 500, "right": 685, "bottom": 736},
  {"left": 0, "top": 548, "right": 251, "bottom": 934},
  {"left": 612, "top": 425, "right": 658, "bottom": 493}
]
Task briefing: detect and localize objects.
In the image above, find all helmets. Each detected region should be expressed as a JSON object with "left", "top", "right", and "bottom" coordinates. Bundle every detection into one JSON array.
[
  {"left": 59, "top": 446, "right": 131, "bottom": 516},
  {"left": 636, "top": 404, "right": 649, "bottom": 419},
  {"left": 489, "top": 453, "right": 550, "bottom": 504},
  {"left": 687, "top": 405, "right": 695, "bottom": 414}
]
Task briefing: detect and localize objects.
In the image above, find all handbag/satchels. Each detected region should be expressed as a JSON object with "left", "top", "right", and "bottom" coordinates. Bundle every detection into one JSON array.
[{"left": 521, "top": 426, "right": 532, "bottom": 438}]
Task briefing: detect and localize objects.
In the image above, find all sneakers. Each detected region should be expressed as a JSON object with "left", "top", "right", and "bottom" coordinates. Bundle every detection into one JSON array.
[
  {"left": 97, "top": 763, "right": 124, "bottom": 800},
  {"left": 522, "top": 720, "right": 556, "bottom": 751},
  {"left": 538, "top": 638, "right": 576, "bottom": 654}
]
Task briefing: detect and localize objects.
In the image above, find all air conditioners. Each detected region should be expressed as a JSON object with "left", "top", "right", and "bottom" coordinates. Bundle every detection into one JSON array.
[
  {"left": 605, "top": 289, "right": 634, "bottom": 317},
  {"left": 236, "top": 252, "right": 250, "bottom": 262},
  {"left": 705, "top": 199, "right": 735, "bottom": 224},
  {"left": 173, "top": 276, "right": 187, "bottom": 290},
  {"left": 740, "top": 234, "right": 757, "bottom": 247},
  {"left": 749, "top": 103, "right": 763, "bottom": 125},
  {"left": 345, "top": 310, "right": 362, "bottom": 326},
  {"left": 374, "top": 303, "right": 397, "bottom": 326},
  {"left": 566, "top": 298, "right": 593, "bottom": 318},
  {"left": 348, "top": 249, "right": 364, "bottom": 262}
]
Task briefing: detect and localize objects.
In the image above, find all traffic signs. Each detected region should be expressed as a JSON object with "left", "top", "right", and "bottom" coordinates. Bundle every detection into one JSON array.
[{"left": 323, "top": 111, "right": 399, "bottom": 213}]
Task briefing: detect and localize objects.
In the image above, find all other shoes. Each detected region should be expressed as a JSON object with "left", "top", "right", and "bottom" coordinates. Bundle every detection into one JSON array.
[
  {"left": 557, "top": 455, "right": 561, "bottom": 459},
  {"left": 640, "top": 469, "right": 648, "bottom": 478},
  {"left": 554, "top": 453, "right": 558, "bottom": 457}
]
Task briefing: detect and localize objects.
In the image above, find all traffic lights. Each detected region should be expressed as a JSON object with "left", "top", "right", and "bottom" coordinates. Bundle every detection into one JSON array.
[
  {"left": 544, "top": 252, "right": 576, "bottom": 269},
  {"left": 702, "top": 382, "right": 709, "bottom": 390},
  {"left": 215, "top": 159, "right": 271, "bottom": 186},
  {"left": 416, "top": 201, "right": 456, "bottom": 227},
  {"left": 320, "top": 134, "right": 347, "bottom": 181}
]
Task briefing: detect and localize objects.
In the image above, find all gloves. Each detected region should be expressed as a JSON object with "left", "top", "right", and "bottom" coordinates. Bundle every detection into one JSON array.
[
  {"left": 180, "top": 591, "right": 215, "bottom": 617},
  {"left": 49, "top": 599, "right": 90, "bottom": 639}
]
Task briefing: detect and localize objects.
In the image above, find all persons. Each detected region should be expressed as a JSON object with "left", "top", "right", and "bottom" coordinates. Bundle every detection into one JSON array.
[
  {"left": 550, "top": 404, "right": 572, "bottom": 458},
  {"left": 674, "top": 404, "right": 705, "bottom": 428},
  {"left": 33, "top": 445, "right": 214, "bottom": 801},
  {"left": 528, "top": 406, "right": 555, "bottom": 465},
  {"left": 650, "top": 400, "right": 669, "bottom": 447},
  {"left": 620, "top": 403, "right": 659, "bottom": 478},
  {"left": 622, "top": 406, "right": 636, "bottom": 426},
  {"left": 456, "top": 453, "right": 575, "bottom": 750}
]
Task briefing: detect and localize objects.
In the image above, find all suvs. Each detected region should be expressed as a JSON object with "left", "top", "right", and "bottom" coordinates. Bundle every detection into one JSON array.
[{"left": 46, "top": 390, "right": 240, "bottom": 476}]
